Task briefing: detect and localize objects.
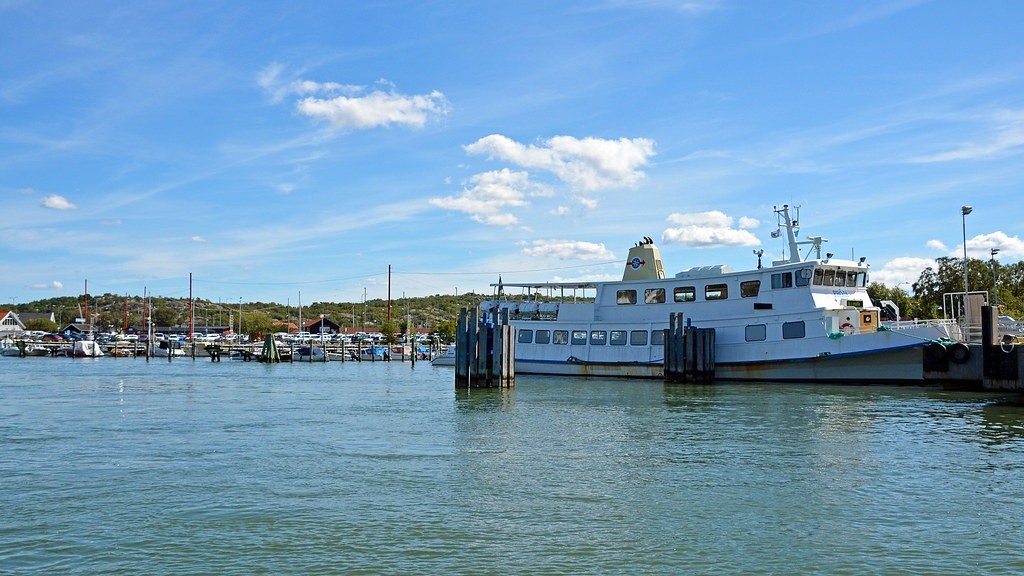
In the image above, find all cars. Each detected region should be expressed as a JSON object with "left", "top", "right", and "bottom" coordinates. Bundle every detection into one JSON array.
[{"left": 26, "top": 331, "right": 444, "bottom": 346}]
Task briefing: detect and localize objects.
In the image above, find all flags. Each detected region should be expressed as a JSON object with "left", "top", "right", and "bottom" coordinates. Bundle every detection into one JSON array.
[{"left": 499, "top": 275, "right": 503, "bottom": 291}]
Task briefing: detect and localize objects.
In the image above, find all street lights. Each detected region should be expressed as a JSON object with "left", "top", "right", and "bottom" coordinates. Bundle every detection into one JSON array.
[
  {"left": 363, "top": 286, "right": 366, "bottom": 333},
  {"left": 990, "top": 246, "right": 1001, "bottom": 308},
  {"left": 961, "top": 205, "right": 973, "bottom": 344},
  {"left": 239, "top": 297, "right": 243, "bottom": 344}
]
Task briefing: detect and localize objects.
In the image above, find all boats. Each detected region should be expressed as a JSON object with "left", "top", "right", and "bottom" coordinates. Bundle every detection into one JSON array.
[
  {"left": 3, "top": 346, "right": 49, "bottom": 356},
  {"left": 61, "top": 339, "right": 104, "bottom": 357},
  {"left": 479, "top": 203, "right": 964, "bottom": 385},
  {"left": 292, "top": 345, "right": 326, "bottom": 362},
  {"left": 431, "top": 341, "right": 457, "bottom": 365},
  {"left": 360, "top": 345, "right": 386, "bottom": 362},
  {"left": 110, "top": 348, "right": 134, "bottom": 357}
]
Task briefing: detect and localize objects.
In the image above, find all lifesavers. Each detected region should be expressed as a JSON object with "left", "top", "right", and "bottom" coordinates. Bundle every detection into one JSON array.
[{"left": 838, "top": 321, "right": 856, "bottom": 337}]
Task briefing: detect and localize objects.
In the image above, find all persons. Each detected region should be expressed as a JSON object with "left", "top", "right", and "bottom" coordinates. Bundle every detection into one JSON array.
[
  {"left": 537, "top": 305, "right": 540, "bottom": 320},
  {"left": 515, "top": 304, "right": 520, "bottom": 320},
  {"left": 556, "top": 305, "right": 559, "bottom": 320}
]
